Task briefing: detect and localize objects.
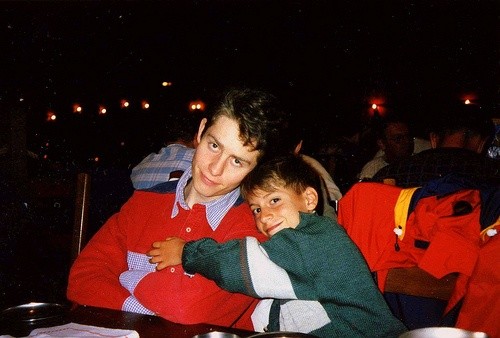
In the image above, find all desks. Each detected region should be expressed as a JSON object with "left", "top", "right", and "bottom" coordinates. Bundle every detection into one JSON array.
[{"left": 0, "top": 297, "right": 260, "bottom": 338}]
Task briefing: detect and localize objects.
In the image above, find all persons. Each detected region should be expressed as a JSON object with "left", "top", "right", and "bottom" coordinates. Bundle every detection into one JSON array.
[
  {"left": 129, "top": 113, "right": 199, "bottom": 189},
  {"left": 294, "top": 72, "right": 500, "bottom": 211},
  {"left": 147, "top": 155, "right": 398, "bottom": 338},
  {"left": 66, "top": 88, "right": 289, "bottom": 331}
]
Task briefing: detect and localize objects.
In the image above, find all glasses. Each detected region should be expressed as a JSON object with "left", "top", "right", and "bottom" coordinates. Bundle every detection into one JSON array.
[{"left": 384, "top": 135, "right": 408, "bottom": 144}]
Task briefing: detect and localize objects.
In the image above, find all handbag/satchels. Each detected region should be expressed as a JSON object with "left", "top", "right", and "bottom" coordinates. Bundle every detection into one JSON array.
[{"left": 336, "top": 147, "right": 482, "bottom": 302}]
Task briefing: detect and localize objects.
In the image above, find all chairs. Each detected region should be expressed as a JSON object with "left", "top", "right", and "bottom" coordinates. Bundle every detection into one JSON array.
[{"left": 337, "top": 182, "right": 500, "bottom": 327}]
